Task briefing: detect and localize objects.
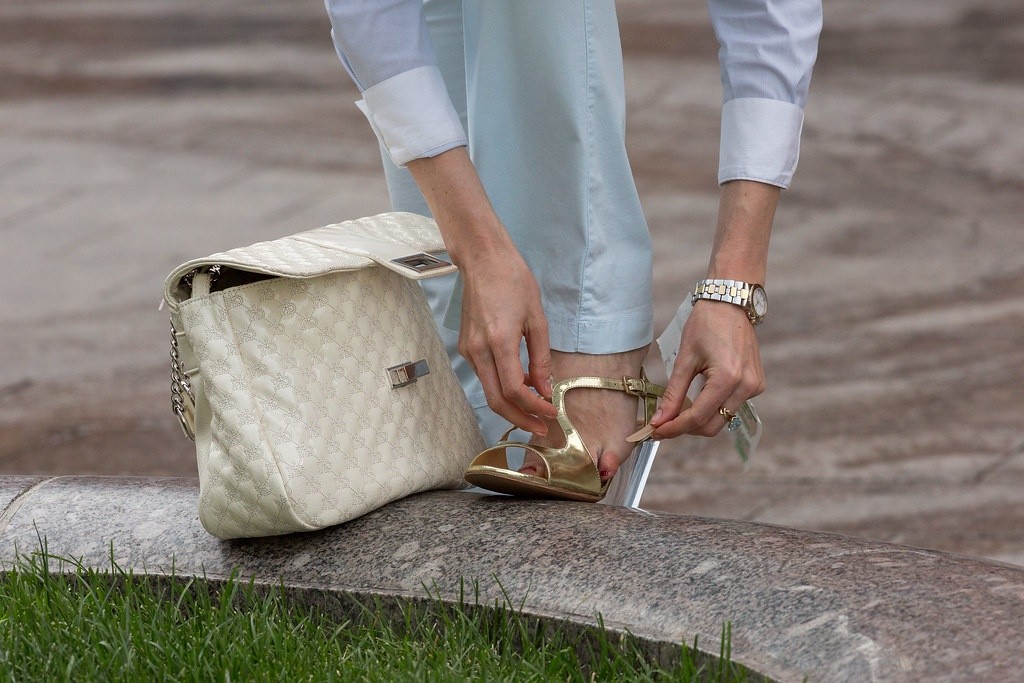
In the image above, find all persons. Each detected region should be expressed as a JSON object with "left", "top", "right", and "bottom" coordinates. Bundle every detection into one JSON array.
[{"left": 324, "top": 0, "right": 824, "bottom": 509}]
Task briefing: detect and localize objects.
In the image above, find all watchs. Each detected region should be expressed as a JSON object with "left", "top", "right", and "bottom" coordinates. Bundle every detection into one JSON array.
[{"left": 692, "top": 279, "right": 768, "bottom": 325}]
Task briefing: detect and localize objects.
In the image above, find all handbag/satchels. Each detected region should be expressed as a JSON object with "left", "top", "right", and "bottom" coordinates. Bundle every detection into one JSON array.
[{"left": 159, "top": 211, "right": 487, "bottom": 541}]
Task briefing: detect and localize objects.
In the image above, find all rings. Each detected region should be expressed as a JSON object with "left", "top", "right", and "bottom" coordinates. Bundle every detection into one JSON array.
[{"left": 720, "top": 405, "right": 742, "bottom": 431}]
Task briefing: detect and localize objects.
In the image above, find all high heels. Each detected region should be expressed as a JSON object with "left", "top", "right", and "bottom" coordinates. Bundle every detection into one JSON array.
[{"left": 463, "top": 361, "right": 693, "bottom": 503}]
